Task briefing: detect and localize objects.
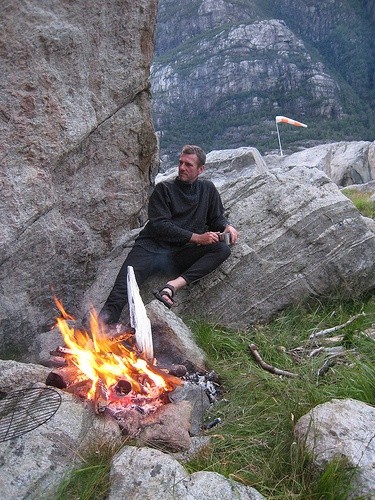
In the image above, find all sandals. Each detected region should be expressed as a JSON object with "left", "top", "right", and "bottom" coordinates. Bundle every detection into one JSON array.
[{"left": 152, "top": 283, "right": 176, "bottom": 309}]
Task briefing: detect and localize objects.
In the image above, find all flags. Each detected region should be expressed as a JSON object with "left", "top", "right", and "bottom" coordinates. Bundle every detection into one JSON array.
[{"left": 276, "top": 115, "right": 307, "bottom": 128}]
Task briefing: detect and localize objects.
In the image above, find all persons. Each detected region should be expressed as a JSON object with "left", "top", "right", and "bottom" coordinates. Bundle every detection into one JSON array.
[{"left": 97, "top": 144, "right": 240, "bottom": 326}]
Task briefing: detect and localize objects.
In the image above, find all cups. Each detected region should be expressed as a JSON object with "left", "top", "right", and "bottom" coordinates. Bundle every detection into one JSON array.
[{"left": 217, "top": 233, "right": 231, "bottom": 246}]
